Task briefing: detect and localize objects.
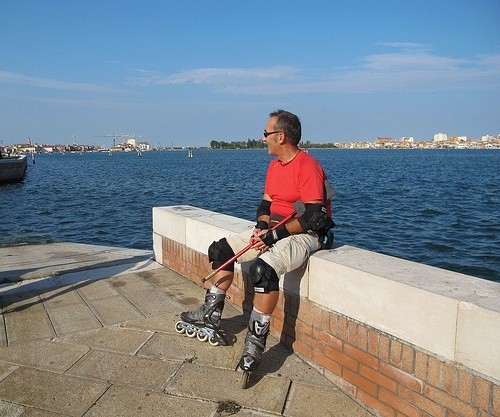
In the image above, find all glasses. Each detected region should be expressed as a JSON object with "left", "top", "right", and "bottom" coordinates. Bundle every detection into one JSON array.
[{"left": 264, "top": 130, "right": 280, "bottom": 137}]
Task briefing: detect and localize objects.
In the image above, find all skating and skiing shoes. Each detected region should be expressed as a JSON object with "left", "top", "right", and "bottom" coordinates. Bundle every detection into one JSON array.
[
  {"left": 235, "top": 306, "right": 272, "bottom": 388},
  {"left": 175, "top": 285, "right": 227, "bottom": 345}
]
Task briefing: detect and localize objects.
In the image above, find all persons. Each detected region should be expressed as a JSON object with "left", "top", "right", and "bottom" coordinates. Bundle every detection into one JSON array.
[{"left": 174, "top": 110, "right": 337, "bottom": 388}]
